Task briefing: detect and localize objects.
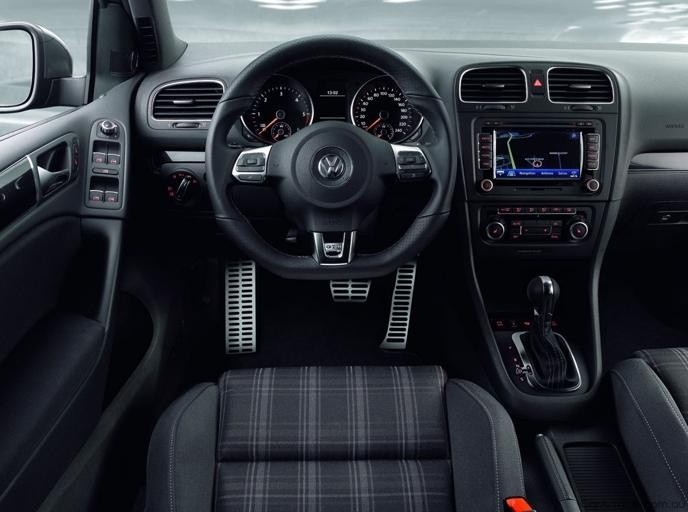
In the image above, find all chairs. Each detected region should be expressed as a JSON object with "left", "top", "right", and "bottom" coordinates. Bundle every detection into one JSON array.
[{"left": 144, "top": 364, "right": 528, "bottom": 511}]
